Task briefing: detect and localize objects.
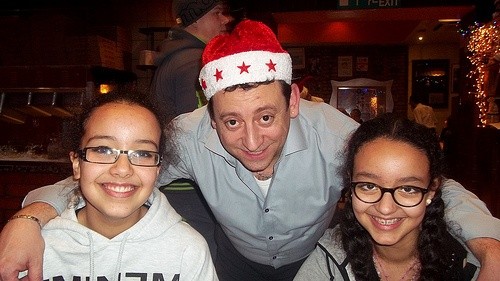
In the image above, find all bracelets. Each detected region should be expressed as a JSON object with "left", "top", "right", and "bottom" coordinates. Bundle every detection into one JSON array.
[{"left": 6, "top": 215, "right": 44, "bottom": 229}]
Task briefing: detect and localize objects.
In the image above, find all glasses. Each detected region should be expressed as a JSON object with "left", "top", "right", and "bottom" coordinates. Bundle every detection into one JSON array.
[
  {"left": 349, "top": 175, "right": 431, "bottom": 208},
  {"left": 76, "top": 146, "right": 163, "bottom": 169}
]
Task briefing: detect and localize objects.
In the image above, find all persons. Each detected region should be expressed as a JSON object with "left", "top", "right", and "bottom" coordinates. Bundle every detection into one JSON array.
[
  {"left": 292, "top": 112, "right": 481, "bottom": 281},
  {"left": 148, "top": 0, "right": 229, "bottom": 123},
  {"left": 0, "top": 19, "right": 500, "bottom": 281},
  {"left": 17, "top": 86, "right": 220, "bottom": 281}
]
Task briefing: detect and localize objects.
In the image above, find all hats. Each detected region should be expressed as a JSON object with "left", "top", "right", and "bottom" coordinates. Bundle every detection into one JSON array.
[
  {"left": 196, "top": 17, "right": 295, "bottom": 100},
  {"left": 174, "top": 0, "right": 224, "bottom": 29}
]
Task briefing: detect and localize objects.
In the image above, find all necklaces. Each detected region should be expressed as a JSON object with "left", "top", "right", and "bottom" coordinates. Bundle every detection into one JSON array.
[
  {"left": 375, "top": 252, "right": 418, "bottom": 281},
  {"left": 256, "top": 171, "right": 272, "bottom": 178}
]
